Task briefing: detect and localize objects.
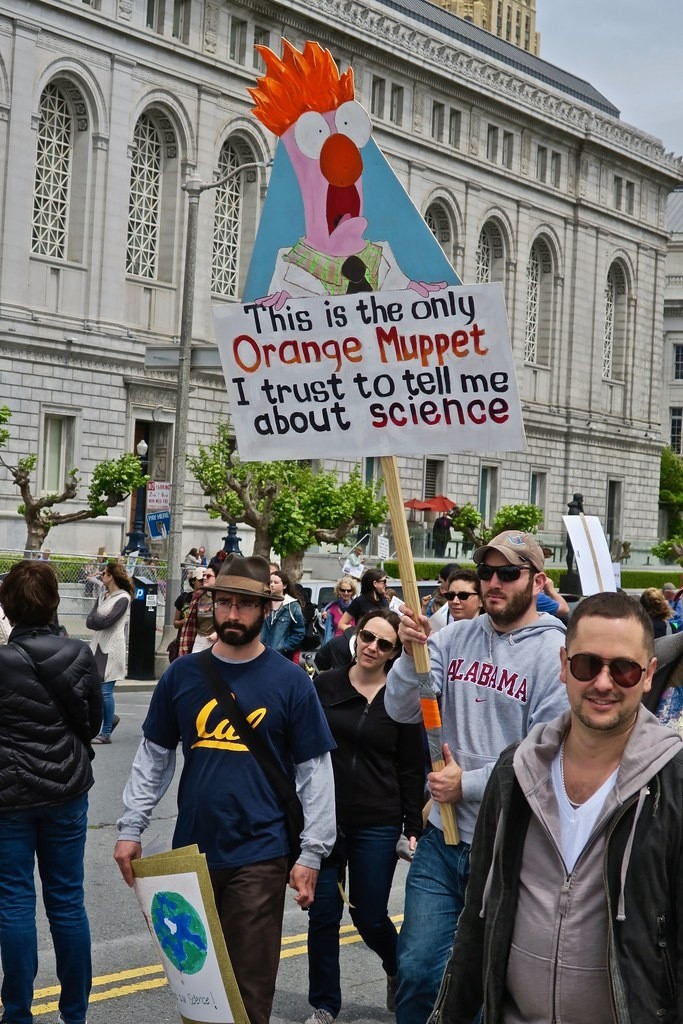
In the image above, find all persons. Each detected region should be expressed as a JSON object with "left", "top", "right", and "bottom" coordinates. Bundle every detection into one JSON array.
[
  {"left": 136, "top": 556, "right": 167, "bottom": 607},
  {"left": 77, "top": 551, "right": 109, "bottom": 597},
  {"left": 565, "top": 493, "right": 586, "bottom": 574},
  {"left": 345, "top": 547, "right": 366, "bottom": 582},
  {"left": 191, "top": 563, "right": 219, "bottom": 654},
  {"left": 640, "top": 537, "right": 683, "bottom": 732},
  {"left": 181, "top": 547, "right": 202, "bottom": 589},
  {"left": 317, "top": 576, "right": 359, "bottom": 647},
  {"left": 433, "top": 511, "right": 455, "bottom": 558},
  {"left": 313, "top": 524, "right": 571, "bottom": 1024},
  {"left": 199, "top": 547, "right": 208, "bottom": 567},
  {"left": 112, "top": 550, "right": 430, "bottom": 1024},
  {"left": 85, "top": 563, "right": 135, "bottom": 745},
  {"left": 39, "top": 548, "right": 51, "bottom": 560},
  {"left": 259, "top": 571, "right": 305, "bottom": 659},
  {"left": 0, "top": 559, "right": 105, "bottom": 1024},
  {"left": 118, "top": 556, "right": 125, "bottom": 566},
  {"left": 427, "top": 591, "right": 683, "bottom": 1024}
]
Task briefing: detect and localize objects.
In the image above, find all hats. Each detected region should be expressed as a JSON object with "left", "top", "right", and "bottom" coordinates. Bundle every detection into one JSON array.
[
  {"left": 475, "top": 530, "right": 545, "bottom": 573},
  {"left": 353, "top": 548, "right": 362, "bottom": 555},
  {"left": 192, "top": 567, "right": 207, "bottom": 580},
  {"left": 199, "top": 553, "right": 284, "bottom": 601}
]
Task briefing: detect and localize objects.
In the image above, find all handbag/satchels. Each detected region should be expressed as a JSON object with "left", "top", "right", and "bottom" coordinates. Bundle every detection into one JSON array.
[{"left": 167, "top": 640, "right": 178, "bottom": 664}]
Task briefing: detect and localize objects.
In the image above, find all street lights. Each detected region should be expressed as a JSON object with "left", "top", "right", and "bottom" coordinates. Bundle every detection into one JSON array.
[
  {"left": 213, "top": 447, "right": 250, "bottom": 561},
  {"left": 119, "top": 438, "right": 156, "bottom": 567}
]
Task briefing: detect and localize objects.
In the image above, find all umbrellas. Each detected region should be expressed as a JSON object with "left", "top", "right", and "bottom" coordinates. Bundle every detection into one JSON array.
[
  {"left": 418, "top": 494, "right": 458, "bottom": 518},
  {"left": 404, "top": 498, "right": 436, "bottom": 526}
]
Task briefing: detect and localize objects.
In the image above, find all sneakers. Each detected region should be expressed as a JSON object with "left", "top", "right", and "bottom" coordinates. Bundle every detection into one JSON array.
[
  {"left": 387, "top": 975, "right": 398, "bottom": 1012},
  {"left": 304, "top": 1009, "right": 336, "bottom": 1024}
]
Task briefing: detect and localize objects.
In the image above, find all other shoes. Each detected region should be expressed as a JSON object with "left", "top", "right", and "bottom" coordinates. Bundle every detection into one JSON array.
[
  {"left": 111, "top": 715, "right": 121, "bottom": 733},
  {"left": 89, "top": 737, "right": 112, "bottom": 744}
]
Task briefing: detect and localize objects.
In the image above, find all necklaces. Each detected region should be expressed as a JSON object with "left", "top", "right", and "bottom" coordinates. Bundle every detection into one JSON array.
[
  {"left": 202, "top": 595, "right": 213, "bottom": 613},
  {"left": 560, "top": 731, "right": 583, "bottom": 807}
]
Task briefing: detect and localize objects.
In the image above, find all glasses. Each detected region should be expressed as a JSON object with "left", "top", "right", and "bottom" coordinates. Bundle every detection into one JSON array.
[
  {"left": 567, "top": 653, "right": 648, "bottom": 688},
  {"left": 203, "top": 574, "right": 216, "bottom": 579},
  {"left": 214, "top": 601, "right": 263, "bottom": 615},
  {"left": 358, "top": 629, "right": 397, "bottom": 653},
  {"left": 378, "top": 579, "right": 387, "bottom": 584},
  {"left": 444, "top": 591, "right": 480, "bottom": 600},
  {"left": 340, "top": 588, "right": 353, "bottom": 592},
  {"left": 102, "top": 571, "right": 110, "bottom": 577},
  {"left": 477, "top": 563, "right": 534, "bottom": 583}
]
